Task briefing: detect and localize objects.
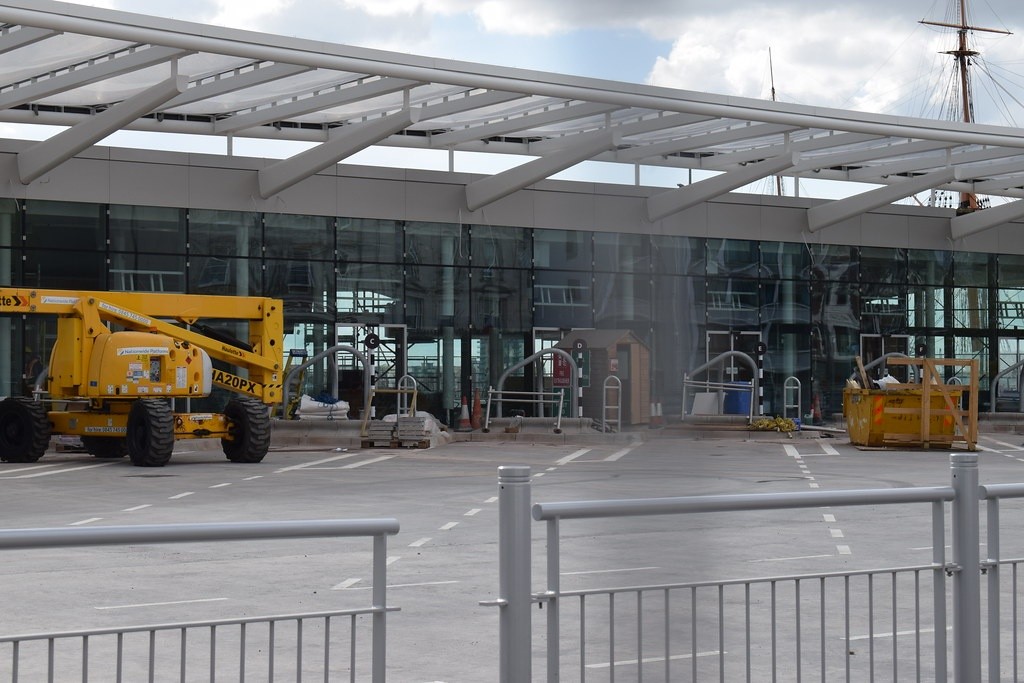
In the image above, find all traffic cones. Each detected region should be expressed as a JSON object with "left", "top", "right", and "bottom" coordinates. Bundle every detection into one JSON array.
[
  {"left": 452, "top": 396, "right": 474, "bottom": 433},
  {"left": 471, "top": 387, "right": 485, "bottom": 430},
  {"left": 811, "top": 391, "right": 826, "bottom": 426}
]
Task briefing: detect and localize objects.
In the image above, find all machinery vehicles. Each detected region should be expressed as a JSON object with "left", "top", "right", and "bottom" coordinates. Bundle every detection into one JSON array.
[{"left": 1, "top": 286, "right": 285, "bottom": 467}]
